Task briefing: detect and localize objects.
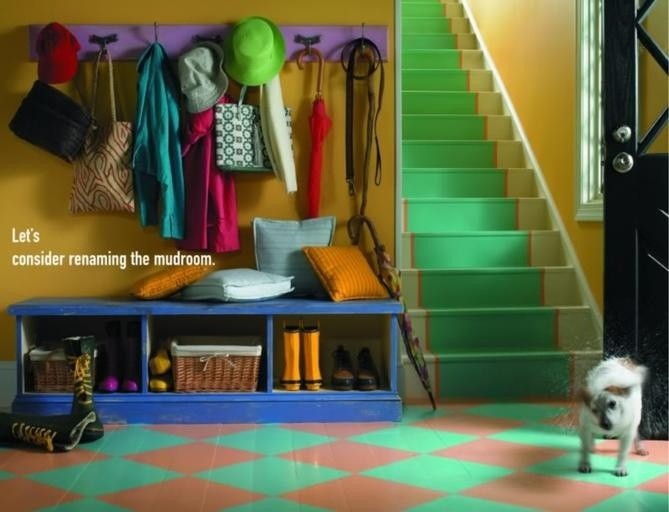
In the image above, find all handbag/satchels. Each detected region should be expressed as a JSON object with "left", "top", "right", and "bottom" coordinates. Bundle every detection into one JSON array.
[
  {"left": 69, "top": 121, "right": 136, "bottom": 215},
  {"left": 214, "top": 103, "right": 292, "bottom": 172},
  {"left": 8, "top": 81, "right": 90, "bottom": 165}
]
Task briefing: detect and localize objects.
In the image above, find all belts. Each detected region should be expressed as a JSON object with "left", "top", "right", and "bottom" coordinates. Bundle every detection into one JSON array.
[{"left": 341, "top": 38, "right": 384, "bottom": 196}]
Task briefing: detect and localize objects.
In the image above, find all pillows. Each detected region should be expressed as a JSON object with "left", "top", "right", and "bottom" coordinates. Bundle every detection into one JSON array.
[{"left": 126, "top": 215, "right": 393, "bottom": 302}]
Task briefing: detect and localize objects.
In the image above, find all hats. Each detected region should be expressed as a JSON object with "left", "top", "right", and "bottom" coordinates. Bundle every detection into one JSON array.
[
  {"left": 37, "top": 22, "right": 81, "bottom": 84},
  {"left": 177, "top": 41, "right": 229, "bottom": 114},
  {"left": 222, "top": 17, "right": 286, "bottom": 87}
]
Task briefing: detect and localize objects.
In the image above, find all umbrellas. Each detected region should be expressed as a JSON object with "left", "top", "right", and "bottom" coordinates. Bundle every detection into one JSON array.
[
  {"left": 346, "top": 213, "right": 437, "bottom": 411},
  {"left": 296, "top": 46, "right": 333, "bottom": 218}
]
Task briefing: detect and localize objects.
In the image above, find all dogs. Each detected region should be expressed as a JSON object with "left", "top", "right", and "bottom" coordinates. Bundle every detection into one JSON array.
[{"left": 577, "top": 354, "right": 651, "bottom": 475}]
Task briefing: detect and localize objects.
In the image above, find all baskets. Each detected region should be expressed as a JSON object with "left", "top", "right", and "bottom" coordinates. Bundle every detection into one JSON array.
[
  {"left": 171, "top": 335, "right": 264, "bottom": 392},
  {"left": 30, "top": 344, "right": 98, "bottom": 393}
]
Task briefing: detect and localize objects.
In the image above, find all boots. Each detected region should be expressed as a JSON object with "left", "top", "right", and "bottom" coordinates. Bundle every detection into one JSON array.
[
  {"left": 332, "top": 346, "right": 355, "bottom": 390},
  {"left": 279, "top": 326, "right": 302, "bottom": 391},
  {"left": 358, "top": 348, "right": 378, "bottom": 390},
  {"left": 0, "top": 411, "right": 96, "bottom": 450},
  {"left": 303, "top": 325, "right": 323, "bottom": 390},
  {"left": 70, "top": 352, "right": 104, "bottom": 437}
]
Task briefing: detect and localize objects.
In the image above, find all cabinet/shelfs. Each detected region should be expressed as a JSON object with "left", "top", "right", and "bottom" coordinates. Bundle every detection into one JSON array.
[{"left": 6, "top": 290, "right": 407, "bottom": 425}]
